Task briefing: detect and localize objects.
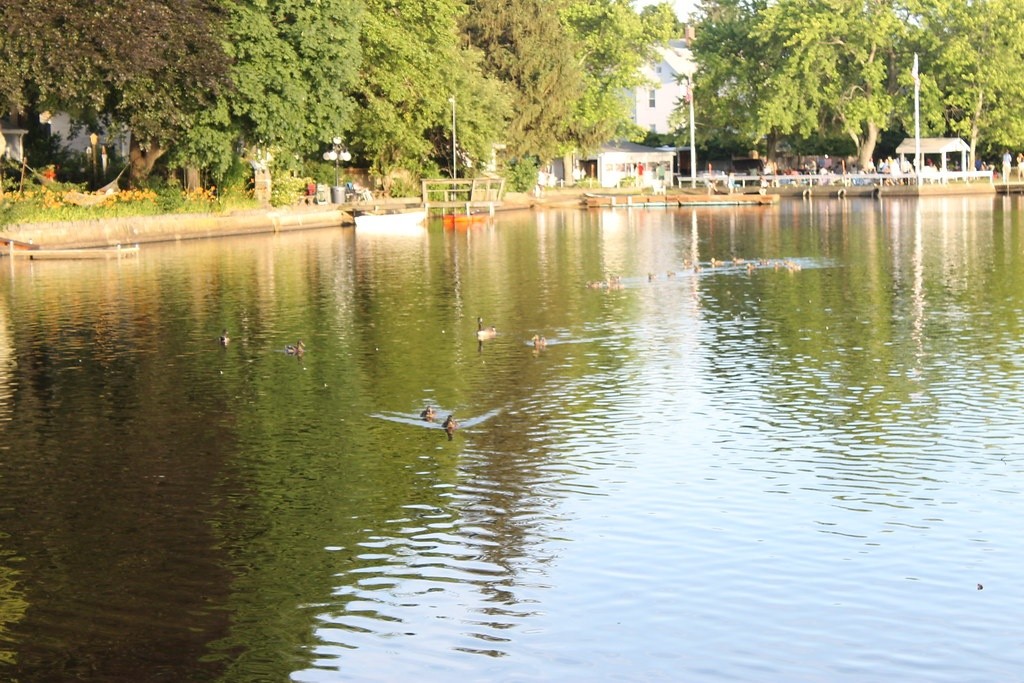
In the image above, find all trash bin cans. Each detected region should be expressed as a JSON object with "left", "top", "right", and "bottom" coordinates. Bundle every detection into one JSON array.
[{"left": 331, "top": 187, "right": 345, "bottom": 204}]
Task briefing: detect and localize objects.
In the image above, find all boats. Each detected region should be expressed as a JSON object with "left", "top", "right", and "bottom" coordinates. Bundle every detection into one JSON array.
[{"left": 580, "top": 190, "right": 780, "bottom": 206}]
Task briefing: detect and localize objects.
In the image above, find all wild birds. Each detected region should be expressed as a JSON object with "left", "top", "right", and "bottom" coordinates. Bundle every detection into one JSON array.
[
  {"left": 286, "top": 340, "right": 306, "bottom": 354},
  {"left": 219, "top": 329, "right": 230, "bottom": 346},
  {"left": 478, "top": 317, "right": 496, "bottom": 342},
  {"left": 532, "top": 334, "right": 546, "bottom": 348},
  {"left": 421, "top": 406, "right": 436, "bottom": 417},
  {"left": 442, "top": 415, "right": 457, "bottom": 427},
  {"left": 586, "top": 256, "right": 801, "bottom": 289}
]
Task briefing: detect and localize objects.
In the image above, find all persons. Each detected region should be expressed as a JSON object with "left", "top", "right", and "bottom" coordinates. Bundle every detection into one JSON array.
[{"left": 637, "top": 149, "right": 1024, "bottom": 196}]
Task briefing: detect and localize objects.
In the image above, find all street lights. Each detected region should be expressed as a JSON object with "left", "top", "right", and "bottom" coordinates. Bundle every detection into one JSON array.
[
  {"left": 89, "top": 132, "right": 99, "bottom": 191},
  {"left": 322, "top": 136, "right": 352, "bottom": 186},
  {"left": 447, "top": 96, "right": 457, "bottom": 203}
]
